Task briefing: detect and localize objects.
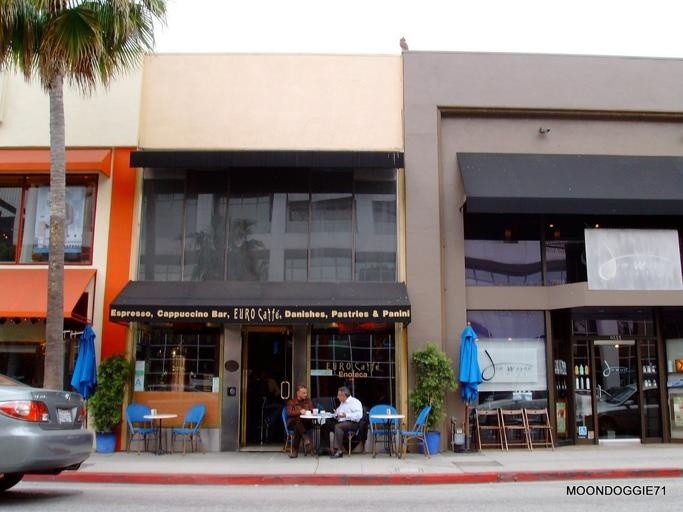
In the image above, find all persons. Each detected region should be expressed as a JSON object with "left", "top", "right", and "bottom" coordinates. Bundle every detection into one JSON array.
[
  {"left": 246, "top": 369, "right": 282, "bottom": 448},
  {"left": 284, "top": 384, "right": 316, "bottom": 457},
  {"left": 318, "top": 385, "right": 363, "bottom": 458}
]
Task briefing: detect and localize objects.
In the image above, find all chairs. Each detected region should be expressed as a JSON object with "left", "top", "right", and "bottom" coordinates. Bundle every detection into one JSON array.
[
  {"left": 474, "top": 408, "right": 555, "bottom": 451},
  {"left": 126, "top": 403, "right": 156, "bottom": 453},
  {"left": 172, "top": 404, "right": 206, "bottom": 454},
  {"left": 281, "top": 404, "right": 432, "bottom": 459}
]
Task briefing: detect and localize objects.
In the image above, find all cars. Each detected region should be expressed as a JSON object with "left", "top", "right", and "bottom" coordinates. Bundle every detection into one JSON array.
[
  {"left": 143, "top": 383, "right": 201, "bottom": 392},
  {"left": 0, "top": 366, "right": 101, "bottom": 504},
  {"left": 478, "top": 381, "right": 662, "bottom": 437}
]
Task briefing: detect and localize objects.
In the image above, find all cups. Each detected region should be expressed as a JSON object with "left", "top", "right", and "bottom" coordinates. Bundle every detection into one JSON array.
[
  {"left": 326, "top": 413, "right": 330, "bottom": 416},
  {"left": 151, "top": 408, "right": 158, "bottom": 417},
  {"left": 313, "top": 409, "right": 318, "bottom": 416},
  {"left": 307, "top": 411, "right": 311, "bottom": 416},
  {"left": 320, "top": 411, "right": 325, "bottom": 416}
]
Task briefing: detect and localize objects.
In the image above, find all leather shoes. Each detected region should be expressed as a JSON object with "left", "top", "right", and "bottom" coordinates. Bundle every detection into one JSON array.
[
  {"left": 311, "top": 449, "right": 332, "bottom": 457},
  {"left": 328, "top": 449, "right": 343, "bottom": 458},
  {"left": 288, "top": 451, "right": 299, "bottom": 458},
  {"left": 303, "top": 439, "right": 311, "bottom": 447}
]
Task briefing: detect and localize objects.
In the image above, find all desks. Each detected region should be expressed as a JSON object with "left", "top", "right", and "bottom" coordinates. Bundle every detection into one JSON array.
[{"left": 142, "top": 414, "right": 178, "bottom": 455}]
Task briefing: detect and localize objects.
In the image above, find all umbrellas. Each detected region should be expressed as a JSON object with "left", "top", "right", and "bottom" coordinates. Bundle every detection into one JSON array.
[
  {"left": 69, "top": 322, "right": 97, "bottom": 429},
  {"left": 457, "top": 321, "right": 482, "bottom": 448}
]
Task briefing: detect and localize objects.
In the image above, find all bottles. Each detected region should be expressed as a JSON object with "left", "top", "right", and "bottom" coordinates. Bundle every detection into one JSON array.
[
  {"left": 575, "top": 377, "right": 592, "bottom": 389},
  {"left": 386, "top": 409, "right": 391, "bottom": 416},
  {"left": 643, "top": 378, "right": 657, "bottom": 387},
  {"left": 642, "top": 365, "right": 656, "bottom": 373},
  {"left": 575, "top": 364, "right": 589, "bottom": 375}
]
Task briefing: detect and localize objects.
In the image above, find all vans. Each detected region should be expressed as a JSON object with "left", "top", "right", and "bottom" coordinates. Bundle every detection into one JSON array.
[{"left": 160, "top": 372, "right": 214, "bottom": 393}]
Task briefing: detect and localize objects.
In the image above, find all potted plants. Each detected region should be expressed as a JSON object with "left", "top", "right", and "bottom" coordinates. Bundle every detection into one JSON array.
[
  {"left": 408, "top": 343, "right": 458, "bottom": 455},
  {"left": 87, "top": 352, "right": 130, "bottom": 453}
]
